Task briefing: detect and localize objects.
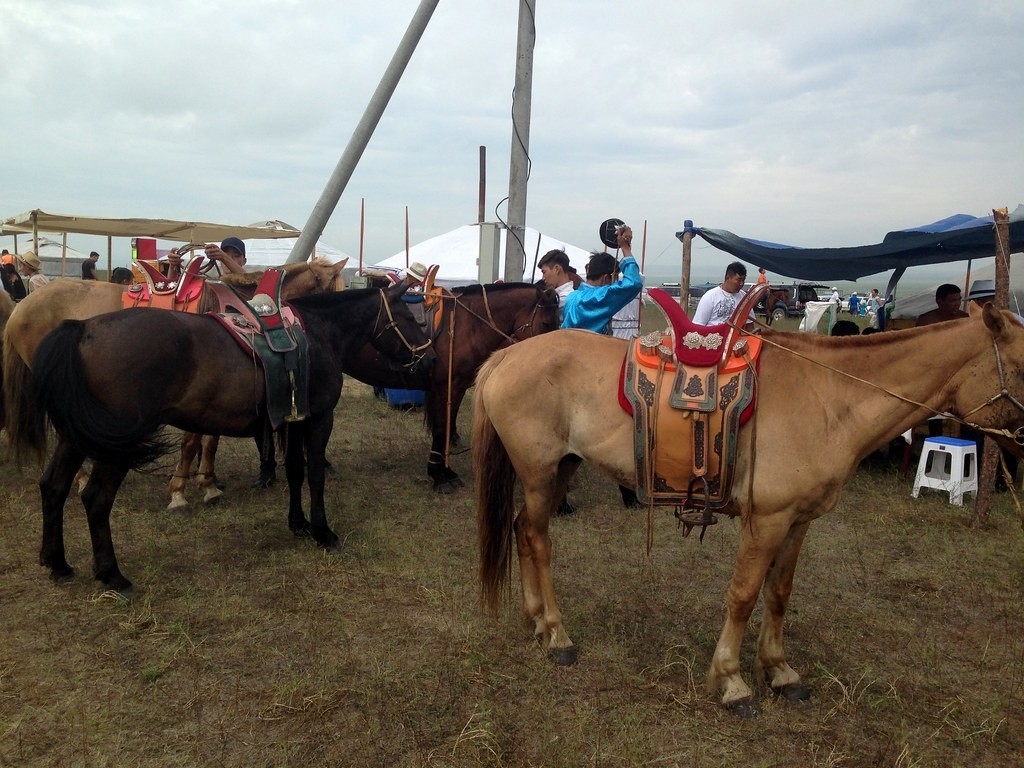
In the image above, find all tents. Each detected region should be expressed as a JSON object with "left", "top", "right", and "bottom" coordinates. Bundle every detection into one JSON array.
[
  {"left": 356, "top": 221, "right": 644, "bottom": 341},
  {"left": 159, "top": 224, "right": 369, "bottom": 287},
  {"left": 891, "top": 252, "right": 1024, "bottom": 319}
]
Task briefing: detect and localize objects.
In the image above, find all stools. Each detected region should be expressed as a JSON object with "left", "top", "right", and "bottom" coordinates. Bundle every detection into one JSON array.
[{"left": 910, "top": 436, "right": 979, "bottom": 506}]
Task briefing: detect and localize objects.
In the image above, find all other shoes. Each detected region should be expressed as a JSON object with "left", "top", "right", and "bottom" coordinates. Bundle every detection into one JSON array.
[
  {"left": 256, "top": 462, "right": 276, "bottom": 488},
  {"left": 216, "top": 481, "right": 225, "bottom": 488},
  {"left": 623, "top": 496, "right": 640, "bottom": 507},
  {"left": 557, "top": 501, "right": 578, "bottom": 514}
]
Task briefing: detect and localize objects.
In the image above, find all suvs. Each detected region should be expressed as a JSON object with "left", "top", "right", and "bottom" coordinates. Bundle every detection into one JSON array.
[{"left": 745, "top": 283, "right": 831, "bottom": 322}]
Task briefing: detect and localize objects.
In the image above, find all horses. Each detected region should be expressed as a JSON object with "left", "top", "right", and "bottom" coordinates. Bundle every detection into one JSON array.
[
  {"left": 1, "top": 245, "right": 560, "bottom": 601},
  {"left": 472, "top": 297, "right": 1024, "bottom": 718}
]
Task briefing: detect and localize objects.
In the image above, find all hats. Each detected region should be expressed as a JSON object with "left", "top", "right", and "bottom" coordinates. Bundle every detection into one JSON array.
[
  {"left": 221, "top": 237, "right": 245, "bottom": 256},
  {"left": 852, "top": 292, "right": 857, "bottom": 295},
  {"left": 962, "top": 280, "right": 996, "bottom": 300},
  {"left": 831, "top": 287, "right": 837, "bottom": 290},
  {"left": 14, "top": 250, "right": 40, "bottom": 271}
]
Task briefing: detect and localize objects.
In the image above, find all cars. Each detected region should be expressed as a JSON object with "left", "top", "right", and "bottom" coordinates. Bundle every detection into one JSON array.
[
  {"left": 817, "top": 295, "right": 890, "bottom": 312},
  {"left": 645, "top": 282, "right": 757, "bottom": 309}
]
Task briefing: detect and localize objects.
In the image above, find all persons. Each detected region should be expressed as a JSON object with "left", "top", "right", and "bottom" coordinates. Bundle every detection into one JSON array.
[
  {"left": 829, "top": 287, "right": 839, "bottom": 303},
  {"left": 691, "top": 262, "right": 756, "bottom": 330},
  {"left": 833, "top": 319, "right": 907, "bottom": 465},
  {"left": 538, "top": 248, "right": 575, "bottom": 324},
  {"left": 0, "top": 250, "right": 16, "bottom": 269},
  {"left": 848, "top": 289, "right": 883, "bottom": 327},
  {"left": 167, "top": 237, "right": 277, "bottom": 488},
  {"left": 81, "top": 251, "right": 99, "bottom": 281},
  {"left": 112, "top": 267, "right": 135, "bottom": 284},
  {"left": 9, "top": 251, "right": 49, "bottom": 294},
  {"left": 757, "top": 267, "right": 766, "bottom": 308},
  {"left": 555, "top": 225, "right": 643, "bottom": 516},
  {"left": 917, "top": 284, "right": 971, "bottom": 440},
  {"left": 963, "top": 280, "right": 1017, "bottom": 492}
]
